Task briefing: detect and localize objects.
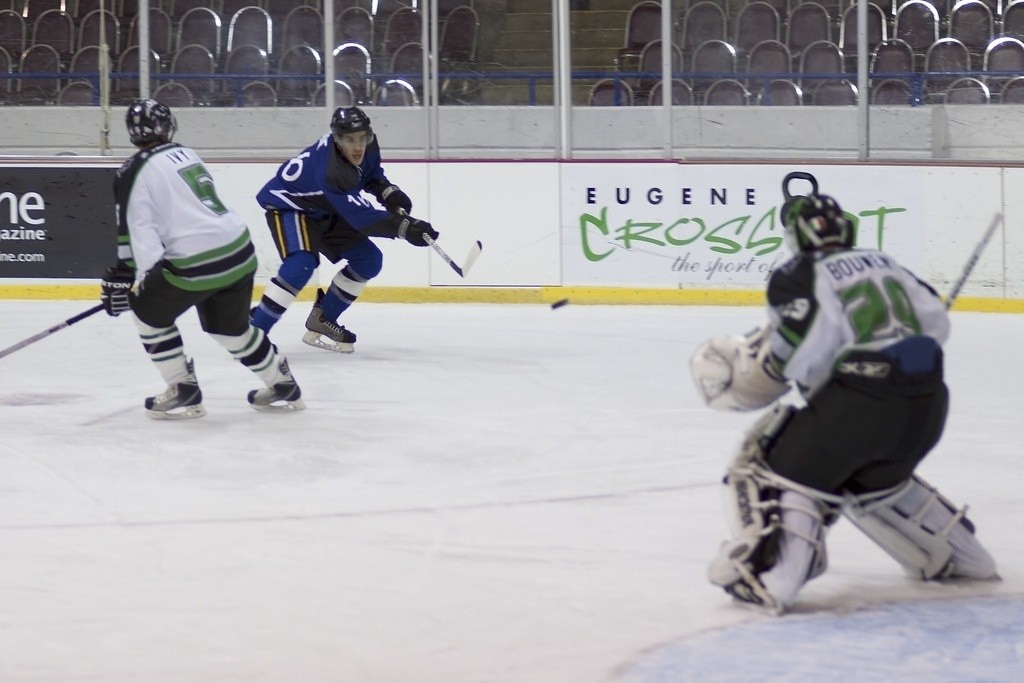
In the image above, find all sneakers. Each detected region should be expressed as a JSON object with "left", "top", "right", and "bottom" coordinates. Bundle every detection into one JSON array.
[
  {"left": 248, "top": 356, "right": 306, "bottom": 412},
  {"left": 734, "top": 596, "right": 783, "bottom": 617},
  {"left": 302, "top": 288, "right": 356, "bottom": 353},
  {"left": 934, "top": 562, "right": 1003, "bottom": 583},
  {"left": 144, "top": 354, "right": 207, "bottom": 419},
  {"left": 249, "top": 307, "right": 257, "bottom": 324}
]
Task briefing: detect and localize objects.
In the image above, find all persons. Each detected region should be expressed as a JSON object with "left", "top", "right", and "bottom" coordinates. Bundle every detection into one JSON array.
[
  {"left": 689, "top": 194, "right": 1002, "bottom": 618},
  {"left": 249, "top": 106, "right": 440, "bottom": 354},
  {"left": 100, "top": 98, "right": 309, "bottom": 421}
]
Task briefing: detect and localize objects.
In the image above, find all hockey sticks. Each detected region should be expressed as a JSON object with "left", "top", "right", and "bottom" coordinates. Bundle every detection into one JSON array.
[
  {"left": 397, "top": 206, "right": 482, "bottom": 277},
  {"left": 946, "top": 211, "right": 1003, "bottom": 310},
  {"left": 0, "top": 304, "right": 105, "bottom": 358}
]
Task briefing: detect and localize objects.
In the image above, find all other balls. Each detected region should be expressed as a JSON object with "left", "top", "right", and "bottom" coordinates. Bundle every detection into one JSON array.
[{"left": 551, "top": 298, "right": 569, "bottom": 309}]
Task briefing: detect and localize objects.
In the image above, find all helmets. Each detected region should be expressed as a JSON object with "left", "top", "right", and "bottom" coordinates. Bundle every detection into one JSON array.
[
  {"left": 126, "top": 99, "right": 171, "bottom": 145},
  {"left": 330, "top": 106, "right": 374, "bottom": 134},
  {"left": 781, "top": 193, "right": 853, "bottom": 254}
]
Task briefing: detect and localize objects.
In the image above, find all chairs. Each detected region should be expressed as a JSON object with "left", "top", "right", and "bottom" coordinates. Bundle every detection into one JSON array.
[
  {"left": 588, "top": 0, "right": 1024, "bottom": 106},
  {"left": 0, "top": 0, "right": 481, "bottom": 107}
]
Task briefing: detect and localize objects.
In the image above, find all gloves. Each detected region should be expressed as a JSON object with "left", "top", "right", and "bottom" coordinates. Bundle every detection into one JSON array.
[
  {"left": 377, "top": 184, "right": 412, "bottom": 216},
  {"left": 385, "top": 211, "right": 439, "bottom": 246},
  {"left": 101, "top": 267, "right": 135, "bottom": 317}
]
